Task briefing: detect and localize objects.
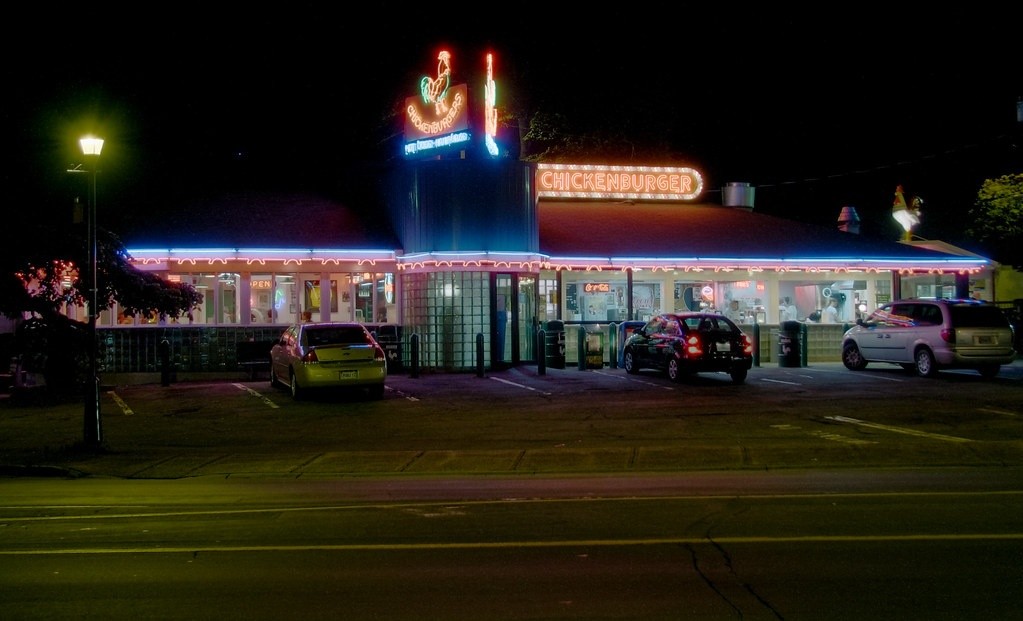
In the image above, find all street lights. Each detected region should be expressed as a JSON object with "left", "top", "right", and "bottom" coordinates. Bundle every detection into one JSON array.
[{"left": 76, "top": 128, "right": 108, "bottom": 448}]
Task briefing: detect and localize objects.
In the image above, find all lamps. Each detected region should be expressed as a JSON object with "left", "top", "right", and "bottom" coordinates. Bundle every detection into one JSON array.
[
  {"left": 218, "top": 273, "right": 234, "bottom": 282},
  {"left": 280, "top": 278, "right": 295, "bottom": 284}
]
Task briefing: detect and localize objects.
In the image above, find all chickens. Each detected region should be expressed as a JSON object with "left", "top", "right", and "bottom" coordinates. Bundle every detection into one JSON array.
[{"left": 892, "top": 185, "right": 923, "bottom": 230}]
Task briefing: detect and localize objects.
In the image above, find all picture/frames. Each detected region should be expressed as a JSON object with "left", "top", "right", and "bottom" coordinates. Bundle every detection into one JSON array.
[
  {"left": 305, "top": 280, "right": 338, "bottom": 313},
  {"left": 605, "top": 294, "right": 614, "bottom": 305}
]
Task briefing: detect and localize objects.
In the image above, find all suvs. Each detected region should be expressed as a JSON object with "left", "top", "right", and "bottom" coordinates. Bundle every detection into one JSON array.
[{"left": 841, "top": 295, "right": 1017, "bottom": 377}]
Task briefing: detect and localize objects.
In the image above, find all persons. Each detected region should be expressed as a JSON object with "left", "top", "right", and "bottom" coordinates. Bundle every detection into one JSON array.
[
  {"left": 377, "top": 306, "right": 387, "bottom": 323},
  {"left": 265, "top": 308, "right": 278, "bottom": 323},
  {"left": 780, "top": 296, "right": 797, "bottom": 321},
  {"left": 823, "top": 297, "right": 847, "bottom": 324},
  {"left": 302, "top": 312, "right": 313, "bottom": 322}
]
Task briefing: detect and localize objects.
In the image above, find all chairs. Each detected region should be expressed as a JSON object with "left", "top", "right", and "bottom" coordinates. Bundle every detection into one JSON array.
[{"left": 697, "top": 321, "right": 711, "bottom": 332}]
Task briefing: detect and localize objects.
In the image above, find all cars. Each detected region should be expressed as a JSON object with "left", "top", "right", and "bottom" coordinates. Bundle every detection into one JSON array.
[
  {"left": 270, "top": 323, "right": 388, "bottom": 400},
  {"left": 622, "top": 313, "right": 752, "bottom": 381}
]
